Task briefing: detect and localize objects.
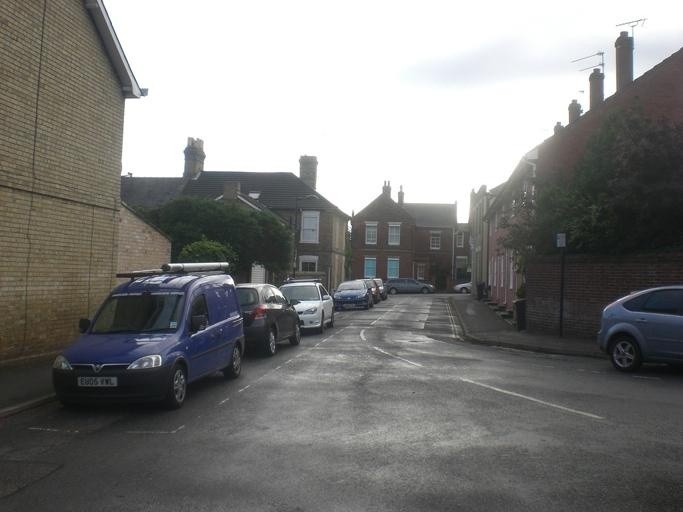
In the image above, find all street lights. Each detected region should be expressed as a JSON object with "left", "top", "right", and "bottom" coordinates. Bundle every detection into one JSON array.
[{"left": 292, "top": 193, "right": 320, "bottom": 278}]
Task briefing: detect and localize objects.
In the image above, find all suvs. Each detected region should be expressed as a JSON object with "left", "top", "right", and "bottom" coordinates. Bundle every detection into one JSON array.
[
  {"left": 276, "top": 278, "right": 336, "bottom": 335},
  {"left": 594, "top": 283, "right": 683, "bottom": 374},
  {"left": 50, "top": 261, "right": 247, "bottom": 411},
  {"left": 384, "top": 277, "right": 436, "bottom": 295}
]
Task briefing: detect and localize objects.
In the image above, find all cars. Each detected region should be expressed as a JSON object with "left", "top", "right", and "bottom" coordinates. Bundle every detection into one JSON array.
[
  {"left": 332, "top": 276, "right": 389, "bottom": 311},
  {"left": 454, "top": 280, "right": 472, "bottom": 294},
  {"left": 231, "top": 281, "right": 305, "bottom": 359}
]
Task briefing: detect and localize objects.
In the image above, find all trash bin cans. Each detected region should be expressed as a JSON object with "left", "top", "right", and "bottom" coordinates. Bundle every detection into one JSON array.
[{"left": 512, "top": 298, "right": 526, "bottom": 330}]
